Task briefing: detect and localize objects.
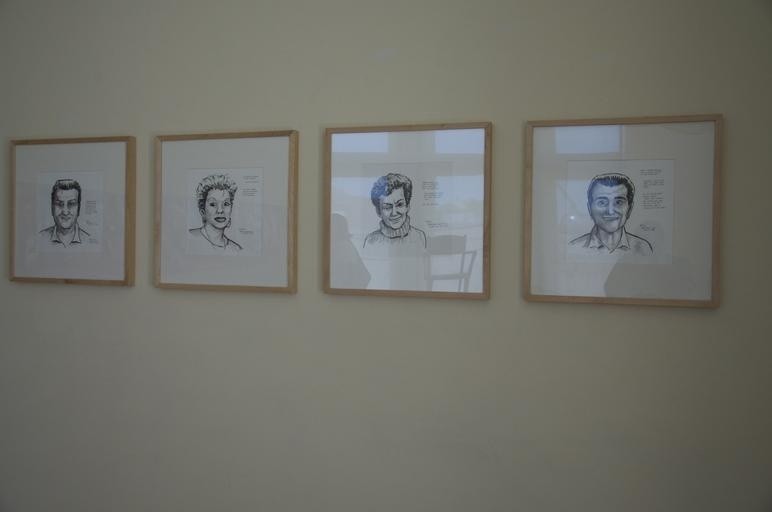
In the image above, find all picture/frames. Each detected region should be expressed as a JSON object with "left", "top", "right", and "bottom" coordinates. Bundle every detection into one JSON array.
[
  {"left": 323, "top": 122, "right": 491, "bottom": 301},
  {"left": 523, "top": 114, "right": 723, "bottom": 310},
  {"left": 9, "top": 136, "right": 136, "bottom": 286},
  {"left": 153, "top": 129, "right": 298, "bottom": 294}
]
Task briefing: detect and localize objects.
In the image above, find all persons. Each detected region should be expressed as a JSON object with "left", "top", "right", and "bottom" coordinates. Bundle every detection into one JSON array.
[
  {"left": 37, "top": 178, "right": 91, "bottom": 248},
  {"left": 189, "top": 174, "right": 242, "bottom": 253},
  {"left": 362, "top": 172, "right": 427, "bottom": 250},
  {"left": 569, "top": 173, "right": 654, "bottom": 255}
]
